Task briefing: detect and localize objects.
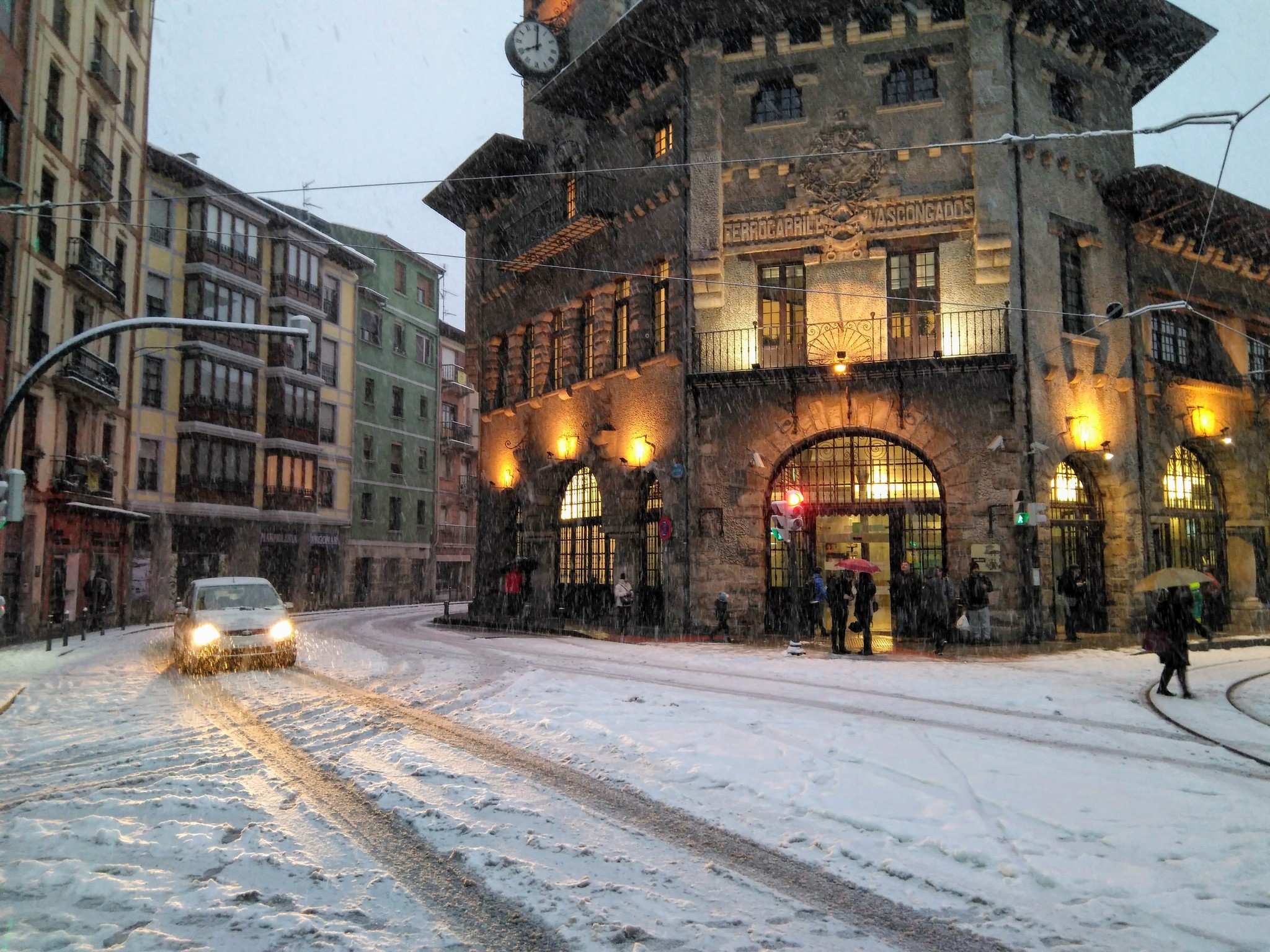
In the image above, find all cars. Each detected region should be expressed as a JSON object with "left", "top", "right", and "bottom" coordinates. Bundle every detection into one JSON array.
[{"left": 174, "top": 576, "right": 297, "bottom": 668}]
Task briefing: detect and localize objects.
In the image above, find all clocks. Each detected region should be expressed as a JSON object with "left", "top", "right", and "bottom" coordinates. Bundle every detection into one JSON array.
[{"left": 514, "top": 21, "right": 559, "bottom": 71}]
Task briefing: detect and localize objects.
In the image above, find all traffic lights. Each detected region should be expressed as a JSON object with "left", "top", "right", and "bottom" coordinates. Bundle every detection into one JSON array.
[{"left": 0, "top": 480, "right": 10, "bottom": 529}]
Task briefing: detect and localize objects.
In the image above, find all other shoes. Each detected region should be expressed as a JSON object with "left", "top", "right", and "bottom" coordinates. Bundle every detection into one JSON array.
[
  {"left": 839, "top": 647, "right": 851, "bottom": 654},
  {"left": 1155, "top": 687, "right": 1177, "bottom": 697},
  {"left": 832, "top": 647, "right": 843, "bottom": 655},
  {"left": 858, "top": 647, "right": 873, "bottom": 656},
  {"left": 932, "top": 639, "right": 947, "bottom": 655},
  {"left": 1183, "top": 692, "right": 1196, "bottom": 699},
  {"left": 820, "top": 632, "right": 830, "bottom": 637},
  {"left": 1067, "top": 636, "right": 1082, "bottom": 642}
]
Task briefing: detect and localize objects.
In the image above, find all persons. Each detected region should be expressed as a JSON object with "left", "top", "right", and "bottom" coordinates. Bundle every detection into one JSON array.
[
  {"left": 614, "top": 573, "right": 634, "bottom": 636},
  {"left": 824, "top": 568, "right": 877, "bottom": 656},
  {"left": 84, "top": 569, "right": 113, "bottom": 635},
  {"left": 920, "top": 565, "right": 957, "bottom": 656},
  {"left": 504, "top": 563, "right": 526, "bottom": 620},
  {"left": 807, "top": 566, "right": 830, "bottom": 637},
  {"left": 709, "top": 591, "right": 735, "bottom": 642},
  {"left": 1155, "top": 585, "right": 1213, "bottom": 699},
  {"left": 1057, "top": 563, "right": 1085, "bottom": 644},
  {"left": 1180, "top": 568, "right": 1228, "bottom": 648},
  {"left": 890, "top": 561, "right": 922, "bottom": 642},
  {"left": 959, "top": 561, "right": 994, "bottom": 648}
]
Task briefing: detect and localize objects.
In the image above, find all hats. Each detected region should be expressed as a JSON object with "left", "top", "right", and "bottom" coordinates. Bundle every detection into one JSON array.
[
  {"left": 1069, "top": 564, "right": 1080, "bottom": 571},
  {"left": 969, "top": 562, "right": 979, "bottom": 570},
  {"left": 718, "top": 591, "right": 730, "bottom": 602}
]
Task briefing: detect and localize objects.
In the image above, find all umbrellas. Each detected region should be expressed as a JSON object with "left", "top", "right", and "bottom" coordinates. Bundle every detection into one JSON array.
[
  {"left": 833, "top": 559, "right": 882, "bottom": 574},
  {"left": 1133, "top": 567, "right": 1214, "bottom": 596},
  {"left": 498, "top": 556, "right": 540, "bottom": 576}
]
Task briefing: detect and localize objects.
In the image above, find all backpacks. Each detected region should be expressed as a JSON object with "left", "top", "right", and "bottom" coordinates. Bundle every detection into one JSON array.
[{"left": 801, "top": 577, "right": 821, "bottom": 602}]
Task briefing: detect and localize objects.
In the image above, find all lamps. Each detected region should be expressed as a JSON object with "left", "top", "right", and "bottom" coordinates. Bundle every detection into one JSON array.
[
  {"left": 547, "top": 451, "right": 564, "bottom": 460},
  {"left": 837, "top": 351, "right": 846, "bottom": 363},
  {"left": 619, "top": 457, "right": 645, "bottom": 468},
  {"left": 1220, "top": 427, "right": 1229, "bottom": 435},
  {"left": 933, "top": 350, "right": 943, "bottom": 358},
  {"left": 488, "top": 481, "right": 505, "bottom": 488},
  {"left": 1100, "top": 440, "right": 1112, "bottom": 450},
  {"left": 751, "top": 363, "right": 761, "bottom": 369}
]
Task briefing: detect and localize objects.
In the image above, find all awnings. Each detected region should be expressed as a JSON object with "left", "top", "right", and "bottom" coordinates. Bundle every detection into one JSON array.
[{"left": 54, "top": 501, "right": 150, "bottom": 523}]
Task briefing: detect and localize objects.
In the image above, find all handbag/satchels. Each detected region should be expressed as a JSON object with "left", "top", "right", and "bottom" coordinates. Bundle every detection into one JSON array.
[
  {"left": 956, "top": 610, "right": 970, "bottom": 631},
  {"left": 873, "top": 601, "right": 879, "bottom": 612},
  {"left": 619, "top": 594, "right": 634, "bottom": 604},
  {"left": 1142, "top": 632, "right": 1168, "bottom": 653}
]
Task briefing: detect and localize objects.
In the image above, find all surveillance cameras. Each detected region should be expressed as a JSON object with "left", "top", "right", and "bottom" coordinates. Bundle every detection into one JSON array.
[
  {"left": 752, "top": 452, "right": 768, "bottom": 468},
  {"left": 642, "top": 461, "right": 656, "bottom": 473},
  {"left": 1035, "top": 441, "right": 1050, "bottom": 452},
  {"left": 986, "top": 435, "right": 1004, "bottom": 452}
]
